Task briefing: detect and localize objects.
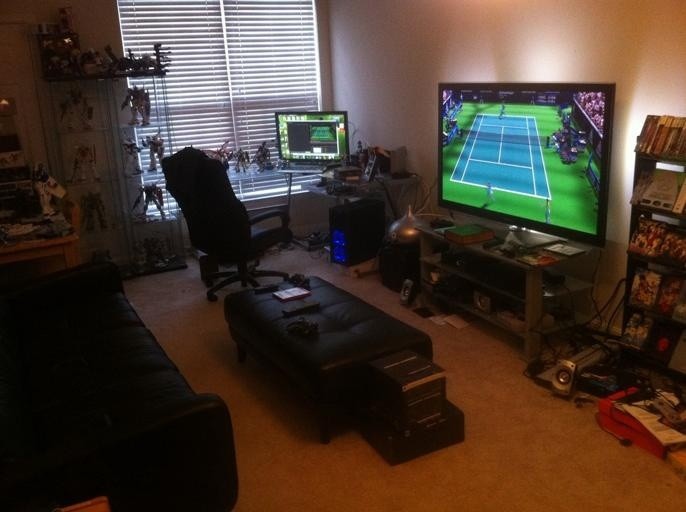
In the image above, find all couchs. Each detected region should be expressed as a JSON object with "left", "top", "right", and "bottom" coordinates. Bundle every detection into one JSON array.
[{"left": 0, "top": 260, "right": 238, "bottom": 512}]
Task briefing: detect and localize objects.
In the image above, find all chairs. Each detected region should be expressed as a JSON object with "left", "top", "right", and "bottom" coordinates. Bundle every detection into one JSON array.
[{"left": 160, "top": 147, "right": 292, "bottom": 301}]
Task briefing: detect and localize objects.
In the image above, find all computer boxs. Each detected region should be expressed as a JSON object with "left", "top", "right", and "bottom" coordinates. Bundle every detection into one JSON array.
[{"left": 329, "top": 198, "right": 386, "bottom": 267}]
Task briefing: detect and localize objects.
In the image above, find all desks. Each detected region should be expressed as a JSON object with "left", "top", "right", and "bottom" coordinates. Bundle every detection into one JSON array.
[
  {"left": 0, "top": 212, "right": 80, "bottom": 269},
  {"left": 278, "top": 163, "right": 421, "bottom": 250}
]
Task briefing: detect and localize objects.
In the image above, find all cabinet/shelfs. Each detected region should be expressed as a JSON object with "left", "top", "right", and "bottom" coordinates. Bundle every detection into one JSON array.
[
  {"left": 621, "top": 136, "right": 686, "bottom": 379},
  {"left": 415, "top": 222, "right": 608, "bottom": 359},
  {"left": 27, "top": 31, "right": 188, "bottom": 279}
]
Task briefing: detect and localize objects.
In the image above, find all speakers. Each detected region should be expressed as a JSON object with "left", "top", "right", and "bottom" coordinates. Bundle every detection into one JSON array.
[
  {"left": 551, "top": 359, "right": 578, "bottom": 400},
  {"left": 399, "top": 278, "right": 417, "bottom": 303}
]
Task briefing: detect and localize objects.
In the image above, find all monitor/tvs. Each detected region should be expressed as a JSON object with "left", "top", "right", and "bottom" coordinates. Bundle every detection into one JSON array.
[
  {"left": 437, "top": 81, "right": 616, "bottom": 248},
  {"left": 275, "top": 111, "right": 350, "bottom": 165}
]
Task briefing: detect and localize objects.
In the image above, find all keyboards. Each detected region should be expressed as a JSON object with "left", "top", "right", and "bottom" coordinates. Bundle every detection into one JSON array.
[{"left": 277, "top": 164, "right": 327, "bottom": 174}]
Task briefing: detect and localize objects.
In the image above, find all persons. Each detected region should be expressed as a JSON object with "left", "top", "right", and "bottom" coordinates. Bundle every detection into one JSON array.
[
  {"left": 202, "top": 140, "right": 270, "bottom": 173},
  {"left": 574, "top": 92, "right": 605, "bottom": 135},
  {"left": 60, "top": 85, "right": 166, "bottom": 232},
  {"left": 545, "top": 199, "right": 551, "bottom": 225},
  {"left": 487, "top": 182, "right": 495, "bottom": 202},
  {"left": 498, "top": 100, "right": 505, "bottom": 119}
]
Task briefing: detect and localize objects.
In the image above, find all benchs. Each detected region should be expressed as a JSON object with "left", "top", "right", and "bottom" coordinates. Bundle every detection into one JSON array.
[{"left": 224, "top": 276, "right": 432, "bottom": 443}]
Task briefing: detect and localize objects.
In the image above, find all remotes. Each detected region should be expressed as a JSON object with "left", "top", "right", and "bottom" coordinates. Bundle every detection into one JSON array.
[{"left": 281, "top": 300, "right": 320, "bottom": 315}]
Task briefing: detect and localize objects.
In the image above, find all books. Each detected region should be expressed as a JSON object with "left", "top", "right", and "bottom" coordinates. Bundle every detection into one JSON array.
[
  {"left": 445, "top": 224, "right": 495, "bottom": 245},
  {"left": 272, "top": 287, "right": 311, "bottom": 303},
  {"left": 334, "top": 166, "right": 363, "bottom": 178},
  {"left": 618, "top": 115, "right": 686, "bottom": 375},
  {"left": 544, "top": 243, "right": 585, "bottom": 257}
]
